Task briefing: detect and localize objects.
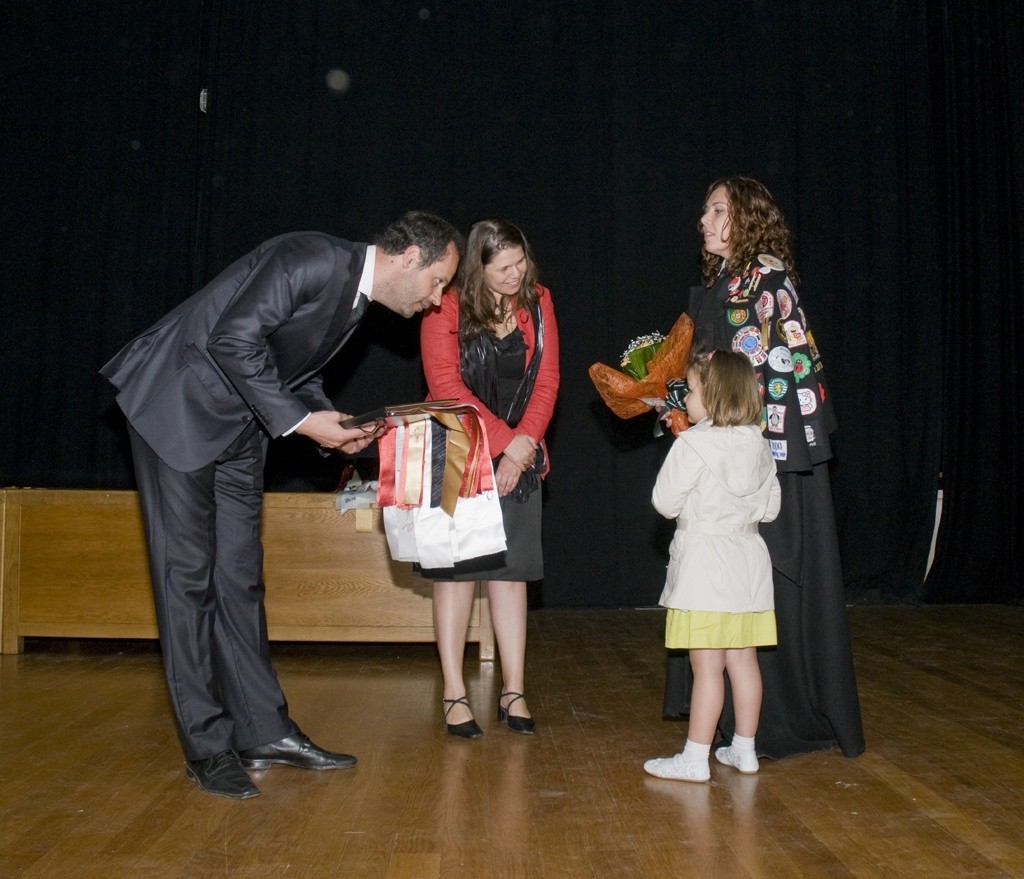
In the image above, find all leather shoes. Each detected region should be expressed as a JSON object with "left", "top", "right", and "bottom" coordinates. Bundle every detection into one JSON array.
[
  {"left": 236, "top": 732, "right": 358, "bottom": 770},
  {"left": 186, "top": 749, "right": 261, "bottom": 800}
]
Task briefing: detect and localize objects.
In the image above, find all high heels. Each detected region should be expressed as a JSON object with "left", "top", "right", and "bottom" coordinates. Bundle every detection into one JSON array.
[
  {"left": 497, "top": 692, "right": 537, "bottom": 734},
  {"left": 442, "top": 696, "right": 484, "bottom": 739}
]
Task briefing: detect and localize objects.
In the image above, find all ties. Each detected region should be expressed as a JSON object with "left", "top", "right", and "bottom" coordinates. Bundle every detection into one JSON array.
[{"left": 341, "top": 292, "right": 368, "bottom": 336}]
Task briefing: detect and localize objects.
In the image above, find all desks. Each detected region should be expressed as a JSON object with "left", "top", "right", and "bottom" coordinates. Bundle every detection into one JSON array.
[{"left": 2, "top": 489, "right": 496, "bottom": 664}]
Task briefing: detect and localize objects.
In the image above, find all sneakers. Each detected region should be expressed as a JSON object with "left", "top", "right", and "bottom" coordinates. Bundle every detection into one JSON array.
[
  {"left": 715, "top": 746, "right": 760, "bottom": 774},
  {"left": 643, "top": 754, "right": 710, "bottom": 783}
]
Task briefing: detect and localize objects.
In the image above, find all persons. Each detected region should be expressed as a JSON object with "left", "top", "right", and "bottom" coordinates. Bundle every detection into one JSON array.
[
  {"left": 413, "top": 219, "right": 559, "bottom": 740},
  {"left": 100, "top": 208, "right": 459, "bottom": 799},
  {"left": 641, "top": 348, "right": 783, "bottom": 782},
  {"left": 654, "top": 175, "right": 864, "bottom": 761}
]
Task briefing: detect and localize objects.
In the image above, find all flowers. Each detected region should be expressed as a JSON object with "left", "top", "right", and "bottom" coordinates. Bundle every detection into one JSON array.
[{"left": 619, "top": 330, "right": 671, "bottom": 385}]
